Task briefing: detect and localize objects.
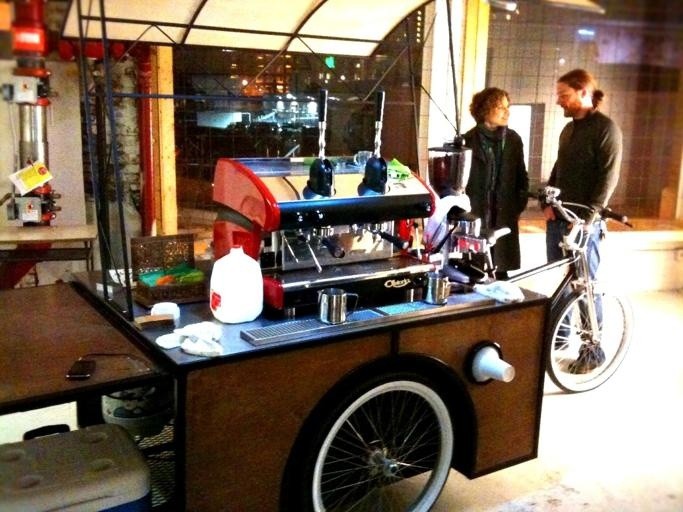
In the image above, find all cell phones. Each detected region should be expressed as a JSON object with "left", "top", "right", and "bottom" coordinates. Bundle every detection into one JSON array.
[{"left": 66, "top": 360, "right": 96, "bottom": 379}]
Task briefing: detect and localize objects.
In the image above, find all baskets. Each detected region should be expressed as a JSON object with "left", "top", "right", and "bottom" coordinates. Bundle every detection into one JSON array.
[{"left": 130, "top": 233, "right": 210, "bottom": 307}]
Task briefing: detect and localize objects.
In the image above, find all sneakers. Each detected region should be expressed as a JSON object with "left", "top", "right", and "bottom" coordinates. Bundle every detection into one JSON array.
[
  {"left": 567, "top": 344, "right": 605, "bottom": 375},
  {"left": 554, "top": 342, "right": 568, "bottom": 350}
]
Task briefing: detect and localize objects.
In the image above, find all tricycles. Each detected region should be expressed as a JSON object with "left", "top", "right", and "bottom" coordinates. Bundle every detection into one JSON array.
[{"left": 0, "top": 0, "right": 631, "bottom": 511}]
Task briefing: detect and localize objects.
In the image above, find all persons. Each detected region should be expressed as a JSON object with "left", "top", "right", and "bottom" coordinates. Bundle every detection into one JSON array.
[
  {"left": 540, "top": 69, "right": 623, "bottom": 374},
  {"left": 454, "top": 87, "right": 528, "bottom": 281}
]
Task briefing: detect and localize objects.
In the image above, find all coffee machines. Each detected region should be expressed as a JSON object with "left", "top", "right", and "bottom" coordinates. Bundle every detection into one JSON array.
[{"left": 423, "top": 136, "right": 474, "bottom": 250}]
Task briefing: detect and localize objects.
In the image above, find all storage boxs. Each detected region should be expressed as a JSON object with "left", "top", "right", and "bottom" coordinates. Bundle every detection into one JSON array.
[{"left": 1, "top": 422, "right": 153, "bottom": 510}]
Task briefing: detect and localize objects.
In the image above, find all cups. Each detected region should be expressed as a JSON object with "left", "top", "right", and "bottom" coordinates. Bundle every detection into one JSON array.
[
  {"left": 318, "top": 289, "right": 358, "bottom": 325},
  {"left": 421, "top": 273, "right": 451, "bottom": 305}
]
